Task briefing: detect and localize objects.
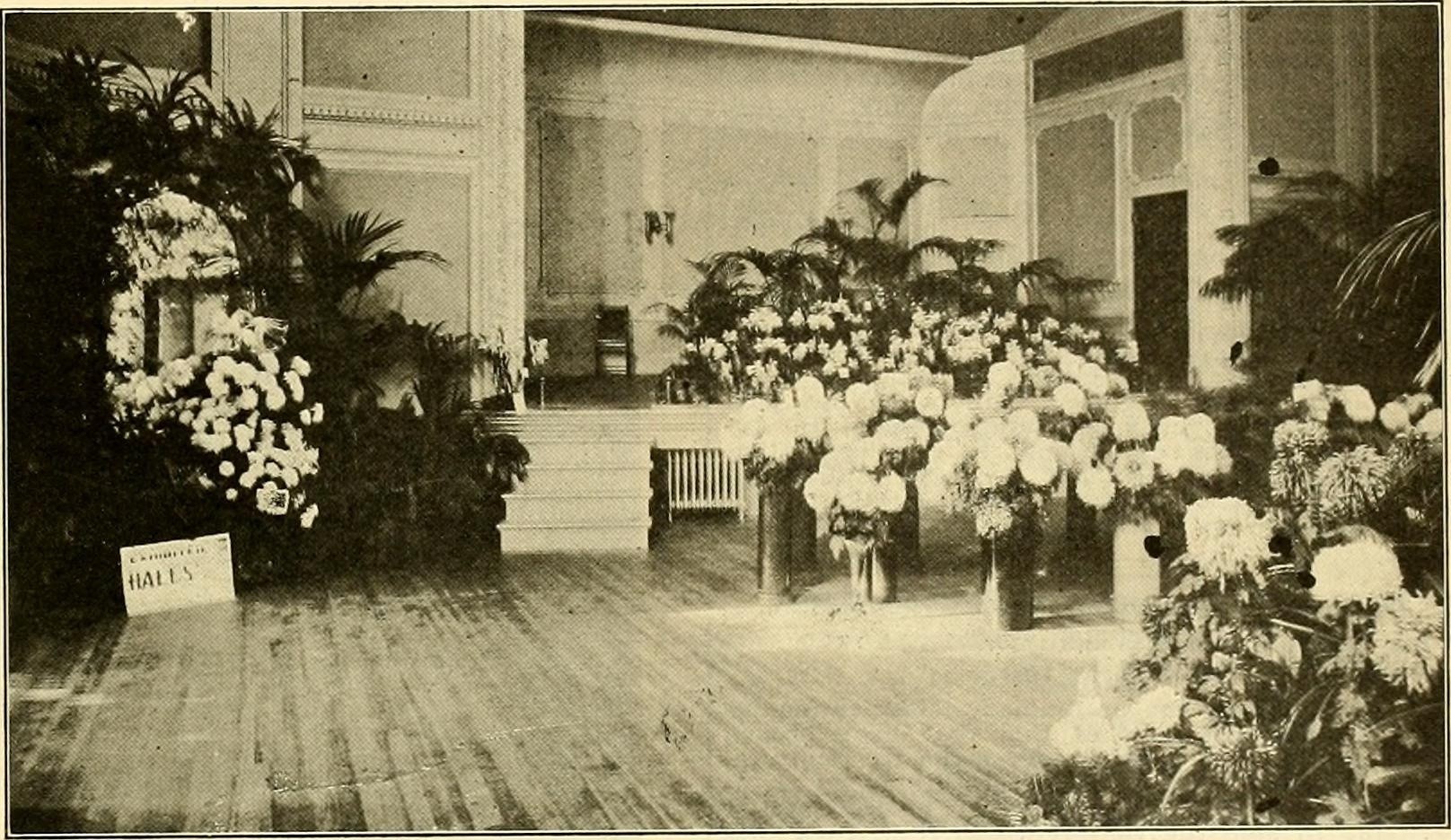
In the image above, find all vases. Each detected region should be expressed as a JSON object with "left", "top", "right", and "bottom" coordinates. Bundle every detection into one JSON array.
[
  {"left": 758, "top": 486, "right": 792, "bottom": 598},
  {"left": 990, "top": 526, "right": 1033, "bottom": 632},
  {"left": 883, "top": 481, "right": 920, "bottom": 562},
  {"left": 850, "top": 540, "right": 900, "bottom": 603},
  {"left": 1066, "top": 471, "right": 1102, "bottom": 558},
  {"left": 788, "top": 490, "right": 824, "bottom": 586}
]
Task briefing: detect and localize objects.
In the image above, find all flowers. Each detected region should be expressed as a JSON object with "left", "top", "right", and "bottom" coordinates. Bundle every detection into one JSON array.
[{"left": 684, "top": 301, "right": 1444, "bottom": 825}]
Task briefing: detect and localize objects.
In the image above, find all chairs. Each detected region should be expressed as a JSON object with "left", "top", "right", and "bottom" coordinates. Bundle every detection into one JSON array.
[{"left": 595, "top": 305, "right": 631, "bottom": 378}]
